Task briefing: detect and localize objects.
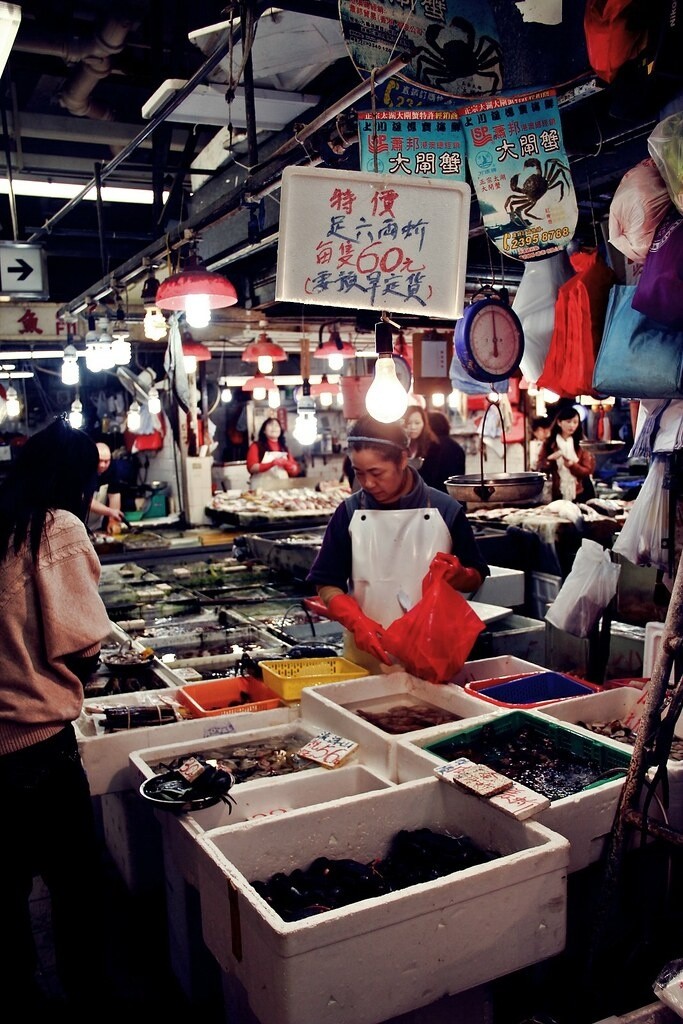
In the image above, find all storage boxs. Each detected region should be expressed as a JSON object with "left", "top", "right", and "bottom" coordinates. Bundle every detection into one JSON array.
[{"left": 70, "top": 498, "right": 683, "bottom": 1024}]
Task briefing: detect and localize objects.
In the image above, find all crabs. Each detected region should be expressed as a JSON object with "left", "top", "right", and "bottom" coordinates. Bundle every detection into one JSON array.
[
  {"left": 504, "top": 158, "right": 572, "bottom": 229},
  {"left": 178, "top": 761, "right": 237, "bottom": 815},
  {"left": 412, "top": 16, "right": 502, "bottom": 96}
]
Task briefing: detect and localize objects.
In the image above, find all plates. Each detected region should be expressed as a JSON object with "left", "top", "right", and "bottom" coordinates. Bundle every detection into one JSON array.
[{"left": 138, "top": 768, "right": 236, "bottom": 814}]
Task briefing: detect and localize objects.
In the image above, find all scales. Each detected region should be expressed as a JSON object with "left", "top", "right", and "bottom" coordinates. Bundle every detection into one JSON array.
[
  {"left": 140, "top": 480, "right": 167, "bottom": 517},
  {"left": 441, "top": 287, "right": 544, "bottom": 504}
]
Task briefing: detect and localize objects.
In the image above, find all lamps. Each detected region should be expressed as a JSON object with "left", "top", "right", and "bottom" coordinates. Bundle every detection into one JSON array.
[
  {"left": 156, "top": 240, "right": 238, "bottom": 328},
  {"left": 182, "top": 344, "right": 211, "bottom": 374},
  {"left": 311, "top": 374, "right": 339, "bottom": 406},
  {"left": 242, "top": 369, "right": 275, "bottom": 400},
  {"left": 314, "top": 323, "right": 356, "bottom": 370},
  {"left": 242, "top": 330, "right": 287, "bottom": 373}
]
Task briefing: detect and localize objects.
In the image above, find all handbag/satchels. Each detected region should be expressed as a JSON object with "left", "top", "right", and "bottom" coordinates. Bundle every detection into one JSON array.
[
  {"left": 584, "top": 0, "right": 683, "bottom": 83},
  {"left": 381, "top": 561, "right": 487, "bottom": 684},
  {"left": 614, "top": 457, "right": 667, "bottom": 566},
  {"left": 509, "top": 112, "right": 683, "bottom": 399},
  {"left": 544, "top": 537, "right": 621, "bottom": 636}
]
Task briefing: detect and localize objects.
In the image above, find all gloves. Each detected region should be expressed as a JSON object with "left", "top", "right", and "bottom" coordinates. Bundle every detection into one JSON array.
[
  {"left": 259, "top": 458, "right": 284, "bottom": 472},
  {"left": 328, "top": 593, "right": 392, "bottom": 666},
  {"left": 429, "top": 552, "right": 481, "bottom": 593},
  {"left": 278, "top": 459, "right": 300, "bottom": 477}
]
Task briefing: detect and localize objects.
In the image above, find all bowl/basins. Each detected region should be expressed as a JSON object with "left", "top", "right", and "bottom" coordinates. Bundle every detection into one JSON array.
[
  {"left": 103, "top": 651, "right": 155, "bottom": 675},
  {"left": 124, "top": 511, "right": 143, "bottom": 521}
]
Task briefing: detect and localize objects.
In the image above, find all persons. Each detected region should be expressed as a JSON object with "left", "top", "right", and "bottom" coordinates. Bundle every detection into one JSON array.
[
  {"left": 305, "top": 414, "right": 491, "bottom": 673},
  {"left": 0, "top": 430, "right": 119, "bottom": 1023},
  {"left": 417, "top": 412, "right": 468, "bottom": 514},
  {"left": 534, "top": 418, "right": 551, "bottom": 439},
  {"left": 87, "top": 443, "right": 124, "bottom": 534},
  {"left": 247, "top": 418, "right": 301, "bottom": 484},
  {"left": 402, "top": 406, "right": 434, "bottom": 459},
  {"left": 538, "top": 408, "right": 596, "bottom": 502}
]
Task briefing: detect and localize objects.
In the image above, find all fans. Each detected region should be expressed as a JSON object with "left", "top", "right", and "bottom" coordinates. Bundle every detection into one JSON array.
[{"left": 116, "top": 366, "right": 169, "bottom": 405}]
